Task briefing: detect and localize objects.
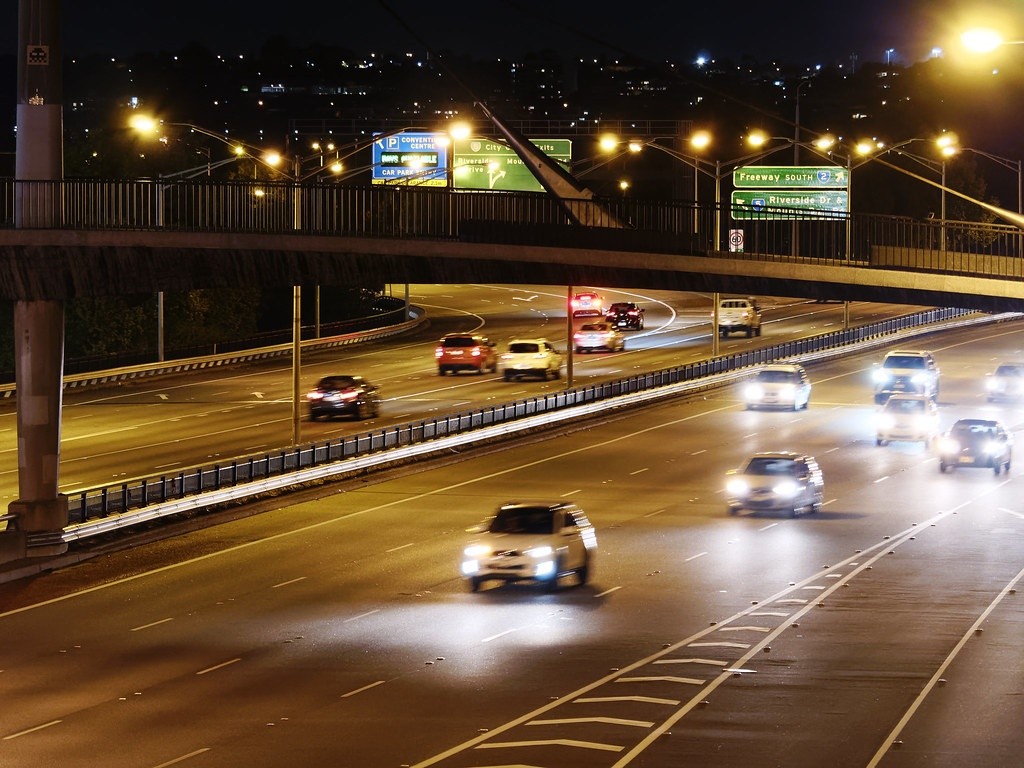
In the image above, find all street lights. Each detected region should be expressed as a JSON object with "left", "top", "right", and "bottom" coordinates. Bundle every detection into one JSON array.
[
  {"left": 128, "top": 116, "right": 500, "bottom": 450},
  {"left": 598, "top": 132, "right": 1022, "bottom": 360}
]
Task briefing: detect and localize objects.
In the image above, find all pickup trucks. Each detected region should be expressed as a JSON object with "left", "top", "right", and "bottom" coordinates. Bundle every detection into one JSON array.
[{"left": 710, "top": 298, "right": 764, "bottom": 338}]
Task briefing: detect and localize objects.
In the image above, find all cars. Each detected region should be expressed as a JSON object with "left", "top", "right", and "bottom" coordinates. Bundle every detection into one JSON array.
[
  {"left": 740, "top": 362, "right": 813, "bottom": 414},
  {"left": 873, "top": 348, "right": 940, "bottom": 408},
  {"left": 303, "top": 373, "right": 383, "bottom": 424},
  {"left": 934, "top": 419, "right": 1014, "bottom": 480},
  {"left": 569, "top": 322, "right": 626, "bottom": 355},
  {"left": 607, "top": 301, "right": 645, "bottom": 330},
  {"left": 873, "top": 393, "right": 935, "bottom": 449},
  {"left": 498, "top": 337, "right": 567, "bottom": 383},
  {"left": 984, "top": 361, "right": 1022, "bottom": 407},
  {"left": 459, "top": 502, "right": 599, "bottom": 595},
  {"left": 722, "top": 451, "right": 825, "bottom": 520},
  {"left": 567, "top": 292, "right": 606, "bottom": 317},
  {"left": 433, "top": 330, "right": 498, "bottom": 377}
]
